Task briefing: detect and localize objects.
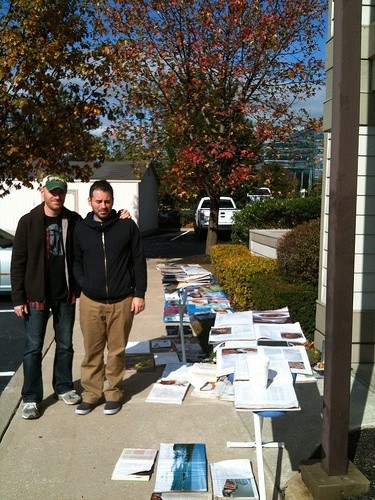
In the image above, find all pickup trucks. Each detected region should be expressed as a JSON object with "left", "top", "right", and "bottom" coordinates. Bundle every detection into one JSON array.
[{"left": 190, "top": 196, "right": 241, "bottom": 234}]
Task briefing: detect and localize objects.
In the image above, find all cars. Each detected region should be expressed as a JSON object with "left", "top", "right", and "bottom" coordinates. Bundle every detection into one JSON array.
[
  {"left": 0, "top": 228, "right": 15, "bottom": 292},
  {"left": 247, "top": 187, "right": 274, "bottom": 205}
]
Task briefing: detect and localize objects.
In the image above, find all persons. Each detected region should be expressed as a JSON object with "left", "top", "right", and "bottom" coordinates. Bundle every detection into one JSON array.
[
  {"left": 10, "top": 174, "right": 133, "bottom": 420},
  {"left": 75, "top": 178, "right": 147, "bottom": 416}
]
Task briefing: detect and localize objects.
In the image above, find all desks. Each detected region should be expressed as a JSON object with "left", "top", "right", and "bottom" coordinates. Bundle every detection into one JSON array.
[
  {"left": 214, "top": 334, "right": 296, "bottom": 500},
  {"left": 162, "top": 274, "right": 235, "bottom": 364}
]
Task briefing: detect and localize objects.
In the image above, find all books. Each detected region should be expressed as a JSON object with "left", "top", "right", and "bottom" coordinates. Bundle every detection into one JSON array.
[
  {"left": 122, "top": 262, "right": 314, "bottom": 409},
  {"left": 110, "top": 443, "right": 260, "bottom": 500}
]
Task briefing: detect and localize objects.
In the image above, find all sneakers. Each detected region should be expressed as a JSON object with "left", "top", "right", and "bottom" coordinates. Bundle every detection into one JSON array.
[
  {"left": 103, "top": 401, "right": 121, "bottom": 414},
  {"left": 21, "top": 402, "right": 40, "bottom": 419},
  {"left": 75, "top": 401, "right": 94, "bottom": 415},
  {"left": 53, "top": 390, "right": 81, "bottom": 405}
]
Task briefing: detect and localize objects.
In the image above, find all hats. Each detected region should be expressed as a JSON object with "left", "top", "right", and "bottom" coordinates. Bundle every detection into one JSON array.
[{"left": 42, "top": 174, "right": 67, "bottom": 192}]
